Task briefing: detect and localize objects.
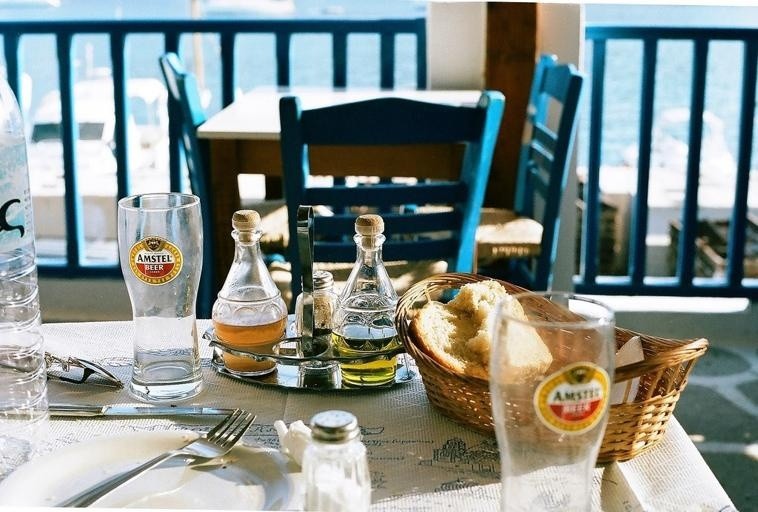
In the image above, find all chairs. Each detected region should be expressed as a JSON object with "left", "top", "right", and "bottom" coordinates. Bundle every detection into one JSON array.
[
  {"left": 415, "top": 52, "right": 585, "bottom": 291},
  {"left": 157, "top": 52, "right": 286, "bottom": 319},
  {"left": 267, "top": 89, "right": 507, "bottom": 314}
]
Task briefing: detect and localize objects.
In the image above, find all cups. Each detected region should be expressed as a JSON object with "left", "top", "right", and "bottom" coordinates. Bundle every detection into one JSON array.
[
  {"left": 116, "top": 188, "right": 208, "bottom": 406},
  {"left": 485, "top": 290, "right": 619, "bottom": 511}
]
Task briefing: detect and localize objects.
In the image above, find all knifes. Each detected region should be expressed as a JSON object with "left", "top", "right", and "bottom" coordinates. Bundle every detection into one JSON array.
[{"left": 0, "top": 396, "right": 249, "bottom": 422}]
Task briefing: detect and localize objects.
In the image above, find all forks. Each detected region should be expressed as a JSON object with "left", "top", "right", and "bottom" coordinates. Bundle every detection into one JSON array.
[{"left": 53, "top": 407, "right": 258, "bottom": 510}]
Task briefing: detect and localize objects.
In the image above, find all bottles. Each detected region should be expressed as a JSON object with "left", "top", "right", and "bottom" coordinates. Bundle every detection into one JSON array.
[
  {"left": 209, "top": 208, "right": 290, "bottom": 379},
  {"left": 330, "top": 211, "right": 402, "bottom": 386},
  {"left": 295, "top": 272, "right": 338, "bottom": 357},
  {"left": 297, "top": 409, "right": 373, "bottom": 512},
  {"left": 0, "top": 34, "right": 65, "bottom": 464}
]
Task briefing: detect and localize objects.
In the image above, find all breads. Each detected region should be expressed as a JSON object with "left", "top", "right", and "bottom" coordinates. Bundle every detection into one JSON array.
[{"left": 407, "top": 278, "right": 555, "bottom": 390}]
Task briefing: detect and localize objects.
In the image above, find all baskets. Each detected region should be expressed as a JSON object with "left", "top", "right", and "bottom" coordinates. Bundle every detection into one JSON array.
[{"left": 393, "top": 272, "right": 711, "bottom": 466}]
